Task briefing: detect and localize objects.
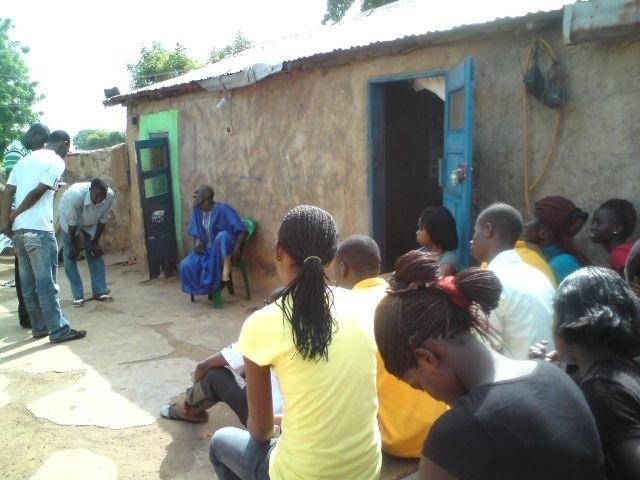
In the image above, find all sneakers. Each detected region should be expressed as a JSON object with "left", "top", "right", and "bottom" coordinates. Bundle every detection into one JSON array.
[
  {"left": 49, "top": 329, "right": 88, "bottom": 344},
  {"left": 32, "top": 327, "right": 49, "bottom": 338}
]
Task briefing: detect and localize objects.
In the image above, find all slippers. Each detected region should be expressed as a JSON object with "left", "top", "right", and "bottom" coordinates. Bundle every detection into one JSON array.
[
  {"left": 92, "top": 294, "right": 114, "bottom": 302},
  {"left": 72, "top": 297, "right": 86, "bottom": 308},
  {"left": 161, "top": 401, "right": 208, "bottom": 425}
]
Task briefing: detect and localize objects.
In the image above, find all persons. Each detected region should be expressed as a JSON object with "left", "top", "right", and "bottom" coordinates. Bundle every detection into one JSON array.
[
  {"left": 589, "top": 198, "right": 638, "bottom": 275},
  {"left": 158, "top": 285, "right": 288, "bottom": 437},
  {"left": 465, "top": 201, "right": 556, "bottom": 361},
  {"left": 0, "top": 130, "right": 87, "bottom": 345},
  {"left": 522, "top": 195, "right": 588, "bottom": 281},
  {"left": 373, "top": 252, "right": 610, "bottom": 480},
  {"left": 209, "top": 204, "right": 384, "bottom": 480},
  {"left": 3, "top": 123, "right": 50, "bottom": 326},
  {"left": 179, "top": 185, "right": 248, "bottom": 302},
  {"left": 527, "top": 266, "right": 640, "bottom": 479},
  {"left": 623, "top": 237, "right": 640, "bottom": 296},
  {"left": 415, "top": 206, "right": 467, "bottom": 277},
  {"left": 333, "top": 233, "right": 449, "bottom": 460},
  {"left": 59, "top": 177, "right": 115, "bottom": 307}
]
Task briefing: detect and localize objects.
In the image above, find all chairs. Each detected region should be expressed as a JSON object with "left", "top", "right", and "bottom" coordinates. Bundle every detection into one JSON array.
[{"left": 190, "top": 217, "right": 256, "bottom": 308}]
